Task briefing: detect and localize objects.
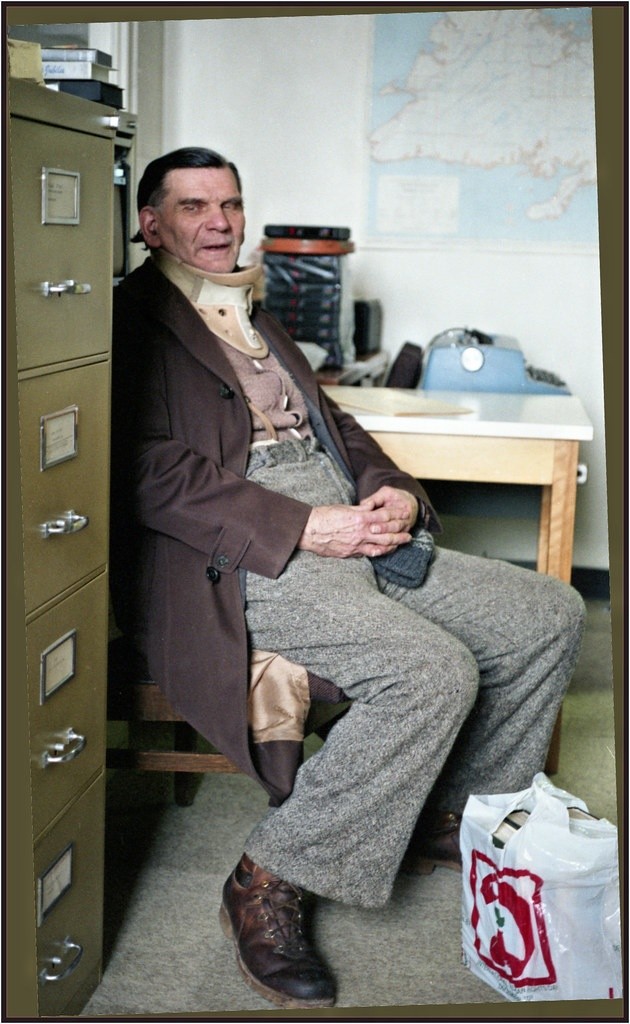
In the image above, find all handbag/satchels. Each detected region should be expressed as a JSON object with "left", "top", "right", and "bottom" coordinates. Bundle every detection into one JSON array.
[{"left": 459, "top": 772, "right": 623, "bottom": 1002}]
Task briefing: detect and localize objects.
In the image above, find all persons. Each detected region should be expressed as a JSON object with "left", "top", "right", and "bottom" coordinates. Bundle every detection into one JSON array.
[{"left": 111, "top": 146, "right": 587, "bottom": 1009}]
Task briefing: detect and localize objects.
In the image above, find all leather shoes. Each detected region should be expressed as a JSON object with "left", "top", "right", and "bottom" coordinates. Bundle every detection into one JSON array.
[
  {"left": 401, "top": 804, "right": 462, "bottom": 875},
  {"left": 219, "top": 853, "right": 336, "bottom": 1009}
]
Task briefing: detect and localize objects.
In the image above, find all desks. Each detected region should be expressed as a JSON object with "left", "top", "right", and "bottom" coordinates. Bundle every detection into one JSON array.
[{"left": 321, "top": 383, "right": 593, "bottom": 776}]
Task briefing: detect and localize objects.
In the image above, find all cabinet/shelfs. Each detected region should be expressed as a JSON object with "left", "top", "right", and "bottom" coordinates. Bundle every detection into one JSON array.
[{"left": 7, "top": 82, "right": 120, "bottom": 1014}]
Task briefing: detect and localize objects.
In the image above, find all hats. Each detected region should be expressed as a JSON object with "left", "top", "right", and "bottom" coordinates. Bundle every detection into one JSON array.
[{"left": 368, "top": 527, "right": 434, "bottom": 587}]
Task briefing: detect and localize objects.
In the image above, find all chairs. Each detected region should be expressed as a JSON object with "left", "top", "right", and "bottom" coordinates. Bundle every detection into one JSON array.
[{"left": 105, "top": 629, "right": 245, "bottom": 804}]
